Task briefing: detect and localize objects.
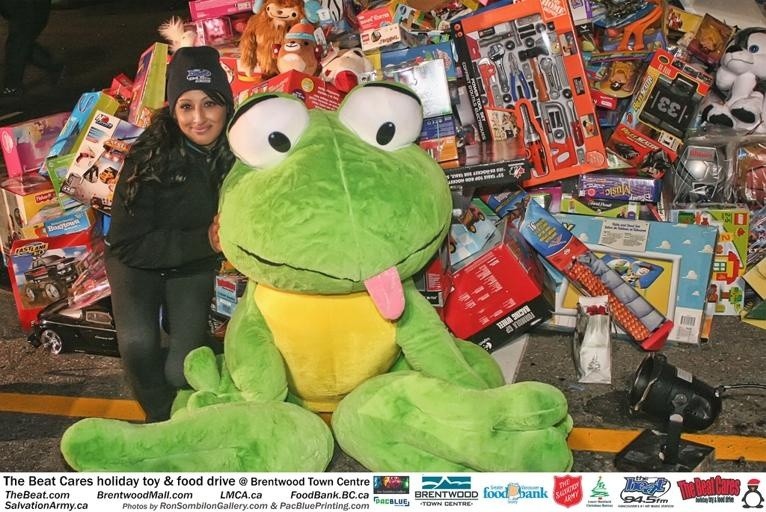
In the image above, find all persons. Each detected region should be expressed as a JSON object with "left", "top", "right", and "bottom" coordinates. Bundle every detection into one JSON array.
[
  {"left": 102, "top": 46, "right": 237, "bottom": 424},
  {"left": 0, "top": 0, "right": 73, "bottom": 121}
]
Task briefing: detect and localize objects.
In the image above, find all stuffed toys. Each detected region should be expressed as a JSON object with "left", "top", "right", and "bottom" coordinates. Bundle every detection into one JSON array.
[
  {"left": 238, "top": 0, "right": 375, "bottom": 93},
  {"left": 699, "top": 27, "right": 766, "bottom": 133},
  {"left": 60, "top": 82, "right": 573, "bottom": 473}
]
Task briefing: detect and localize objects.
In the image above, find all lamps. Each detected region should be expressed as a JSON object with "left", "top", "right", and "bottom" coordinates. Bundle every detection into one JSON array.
[{"left": 615, "top": 352, "right": 725, "bottom": 472}]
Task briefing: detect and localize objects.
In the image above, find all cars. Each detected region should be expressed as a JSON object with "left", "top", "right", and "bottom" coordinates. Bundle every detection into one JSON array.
[{"left": 24, "top": 255, "right": 79, "bottom": 305}]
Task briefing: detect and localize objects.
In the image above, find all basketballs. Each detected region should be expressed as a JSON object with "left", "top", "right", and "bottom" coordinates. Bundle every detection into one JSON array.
[{"left": 745, "top": 148, "right": 765, "bottom": 206}]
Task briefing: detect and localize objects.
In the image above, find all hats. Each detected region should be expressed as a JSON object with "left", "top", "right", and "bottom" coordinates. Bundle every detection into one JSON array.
[{"left": 167, "top": 46, "right": 234, "bottom": 118}]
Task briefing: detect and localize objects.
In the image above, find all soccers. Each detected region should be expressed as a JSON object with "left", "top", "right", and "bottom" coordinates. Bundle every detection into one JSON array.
[{"left": 665, "top": 147, "right": 726, "bottom": 200}]
